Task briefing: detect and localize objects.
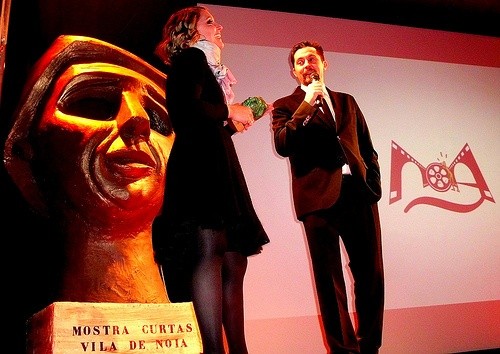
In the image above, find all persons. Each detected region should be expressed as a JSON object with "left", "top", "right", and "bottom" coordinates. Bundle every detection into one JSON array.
[
  {"left": 272, "top": 39, "right": 384, "bottom": 354},
  {"left": 2, "top": 33, "right": 205, "bottom": 354},
  {"left": 148, "top": 5, "right": 270, "bottom": 354}
]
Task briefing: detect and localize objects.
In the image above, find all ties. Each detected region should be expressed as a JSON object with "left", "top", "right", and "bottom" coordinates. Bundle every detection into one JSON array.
[{"left": 316, "top": 95, "right": 348, "bottom": 167}]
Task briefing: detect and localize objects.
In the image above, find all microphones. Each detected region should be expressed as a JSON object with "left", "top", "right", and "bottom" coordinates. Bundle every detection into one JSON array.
[{"left": 310, "top": 73, "right": 323, "bottom": 107}]
